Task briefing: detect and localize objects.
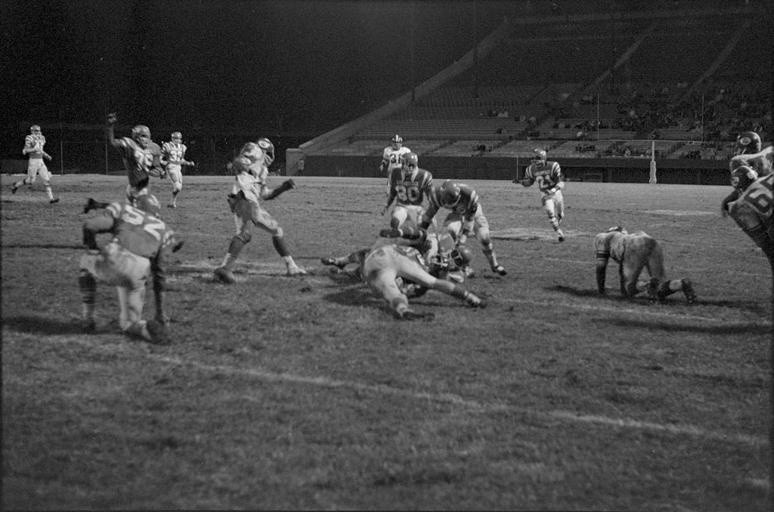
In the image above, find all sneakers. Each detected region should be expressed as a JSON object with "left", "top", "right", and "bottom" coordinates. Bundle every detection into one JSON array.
[
  {"left": 5, "top": 178, "right": 185, "bottom": 214},
  {"left": 646, "top": 274, "right": 661, "bottom": 305},
  {"left": 680, "top": 277, "right": 698, "bottom": 304},
  {"left": 143, "top": 320, "right": 177, "bottom": 347},
  {"left": 553, "top": 213, "right": 570, "bottom": 243},
  {"left": 213, "top": 265, "right": 237, "bottom": 284},
  {"left": 318, "top": 245, "right": 509, "bottom": 325},
  {"left": 73, "top": 314, "right": 96, "bottom": 333},
  {"left": 285, "top": 267, "right": 309, "bottom": 277}
]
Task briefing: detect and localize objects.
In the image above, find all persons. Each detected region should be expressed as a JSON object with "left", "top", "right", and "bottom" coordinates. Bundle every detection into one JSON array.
[
  {"left": 214, "top": 138, "right": 306, "bottom": 284},
  {"left": 11, "top": 125, "right": 60, "bottom": 203},
  {"left": 81, "top": 110, "right": 195, "bottom": 348}
]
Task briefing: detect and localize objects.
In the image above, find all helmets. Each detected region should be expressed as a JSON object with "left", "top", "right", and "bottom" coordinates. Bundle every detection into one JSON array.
[
  {"left": 127, "top": 123, "right": 185, "bottom": 149},
  {"left": 530, "top": 147, "right": 548, "bottom": 168},
  {"left": 391, "top": 134, "right": 466, "bottom": 211},
  {"left": 255, "top": 136, "right": 276, "bottom": 166},
  {"left": 728, "top": 129, "right": 763, "bottom": 190},
  {"left": 29, "top": 123, "right": 42, "bottom": 135}
]
